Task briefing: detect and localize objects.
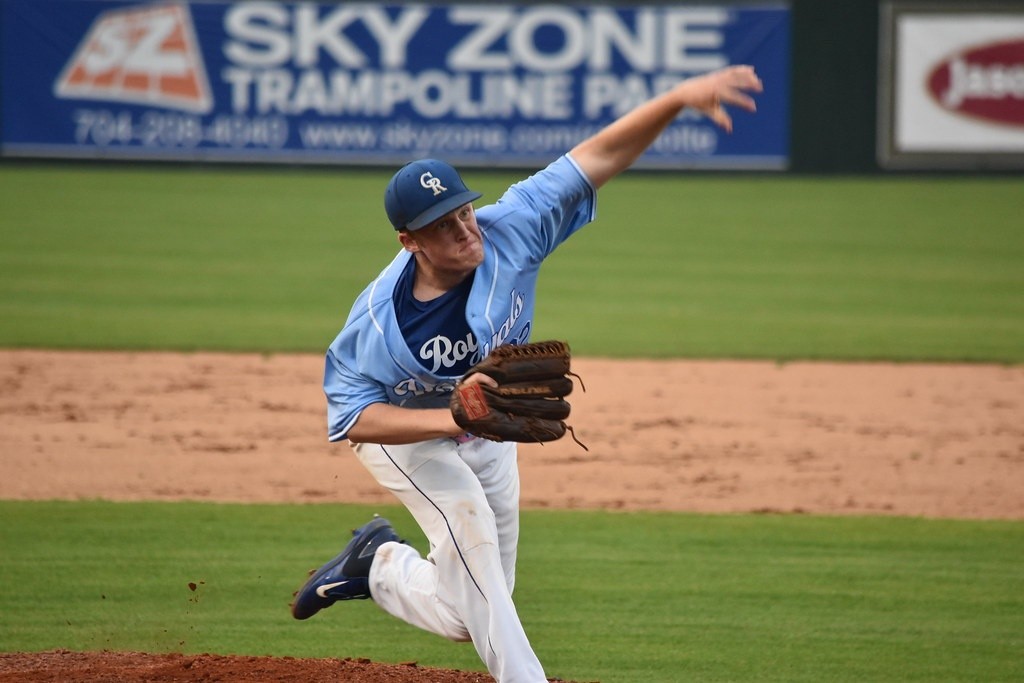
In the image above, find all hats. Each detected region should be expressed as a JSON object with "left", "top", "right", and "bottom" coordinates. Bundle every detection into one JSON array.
[{"left": 383, "top": 158, "right": 484, "bottom": 232}]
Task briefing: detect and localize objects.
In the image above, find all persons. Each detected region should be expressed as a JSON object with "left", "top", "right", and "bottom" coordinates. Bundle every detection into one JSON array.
[{"left": 291, "top": 65, "right": 763, "bottom": 683}]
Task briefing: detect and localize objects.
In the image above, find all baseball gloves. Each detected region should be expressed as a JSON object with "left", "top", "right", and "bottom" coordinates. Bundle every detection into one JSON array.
[{"left": 438, "top": 339, "right": 592, "bottom": 453}]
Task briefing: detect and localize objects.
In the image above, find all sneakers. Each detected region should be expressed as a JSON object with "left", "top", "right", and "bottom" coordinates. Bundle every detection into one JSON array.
[{"left": 289, "top": 512, "right": 410, "bottom": 620}]
